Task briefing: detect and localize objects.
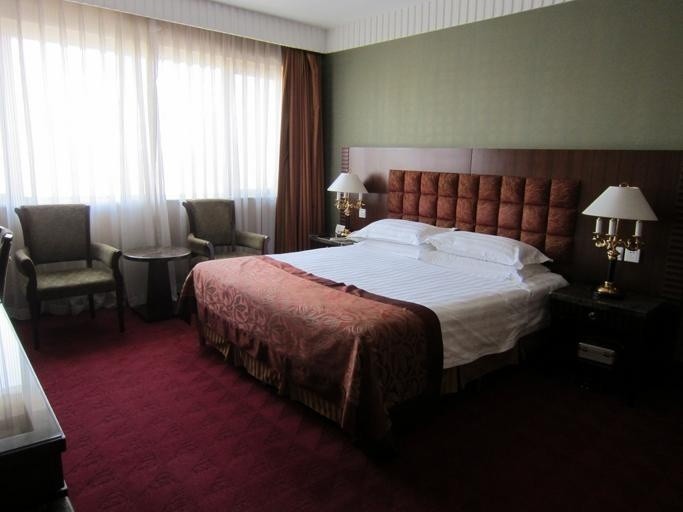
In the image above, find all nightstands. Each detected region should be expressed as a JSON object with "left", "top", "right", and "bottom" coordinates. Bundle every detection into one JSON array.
[
  {"left": 549, "top": 285, "right": 669, "bottom": 409},
  {"left": 307, "top": 231, "right": 357, "bottom": 248}
]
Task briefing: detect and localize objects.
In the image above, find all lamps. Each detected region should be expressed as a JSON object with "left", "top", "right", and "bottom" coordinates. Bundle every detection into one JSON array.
[
  {"left": 326, "top": 173, "right": 370, "bottom": 236},
  {"left": 581, "top": 182, "right": 658, "bottom": 295}
]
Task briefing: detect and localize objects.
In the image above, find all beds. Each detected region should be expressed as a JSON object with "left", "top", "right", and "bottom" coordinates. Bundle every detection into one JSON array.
[{"left": 176, "top": 170, "right": 579, "bottom": 470}]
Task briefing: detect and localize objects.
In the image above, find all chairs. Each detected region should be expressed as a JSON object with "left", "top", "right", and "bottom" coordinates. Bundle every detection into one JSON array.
[
  {"left": 13, "top": 205, "right": 126, "bottom": 350},
  {"left": 0, "top": 224, "right": 13, "bottom": 299},
  {"left": 183, "top": 200, "right": 271, "bottom": 268}
]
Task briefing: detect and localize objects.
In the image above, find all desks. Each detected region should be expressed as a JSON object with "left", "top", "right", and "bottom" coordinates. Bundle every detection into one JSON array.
[
  {"left": 122, "top": 247, "right": 192, "bottom": 323},
  {"left": 0, "top": 303, "right": 74, "bottom": 512}
]
